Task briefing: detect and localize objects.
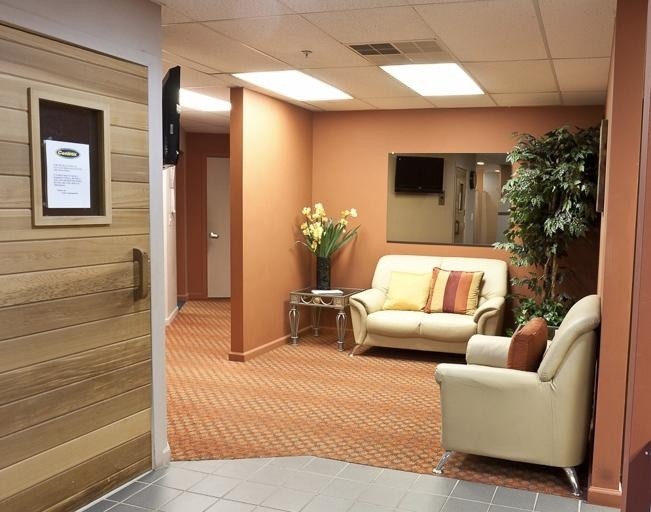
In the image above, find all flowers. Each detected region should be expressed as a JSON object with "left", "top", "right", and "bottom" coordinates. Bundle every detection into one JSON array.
[{"left": 294, "top": 203, "right": 362, "bottom": 256}]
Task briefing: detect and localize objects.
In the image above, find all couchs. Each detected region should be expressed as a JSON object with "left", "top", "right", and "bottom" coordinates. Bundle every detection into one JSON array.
[
  {"left": 434, "top": 294, "right": 601, "bottom": 497},
  {"left": 349, "top": 254, "right": 508, "bottom": 357}
]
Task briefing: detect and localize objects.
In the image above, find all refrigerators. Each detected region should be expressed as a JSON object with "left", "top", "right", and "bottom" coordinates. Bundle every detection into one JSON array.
[{"left": 163, "top": 164, "right": 178, "bottom": 325}]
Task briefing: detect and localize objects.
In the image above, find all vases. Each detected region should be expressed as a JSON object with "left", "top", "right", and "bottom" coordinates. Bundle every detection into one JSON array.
[{"left": 316, "top": 256, "right": 330, "bottom": 290}]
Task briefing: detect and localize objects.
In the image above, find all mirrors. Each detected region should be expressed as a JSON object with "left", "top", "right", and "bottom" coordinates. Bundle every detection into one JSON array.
[{"left": 386, "top": 153, "right": 515, "bottom": 248}]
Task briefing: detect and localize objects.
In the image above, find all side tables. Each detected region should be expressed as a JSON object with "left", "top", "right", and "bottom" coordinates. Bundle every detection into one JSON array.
[{"left": 289, "top": 285, "right": 367, "bottom": 353}]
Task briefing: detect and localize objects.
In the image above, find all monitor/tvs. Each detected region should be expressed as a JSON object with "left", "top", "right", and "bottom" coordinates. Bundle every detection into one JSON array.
[
  {"left": 161, "top": 66, "right": 181, "bottom": 171},
  {"left": 395, "top": 156, "right": 444, "bottom": 194}
]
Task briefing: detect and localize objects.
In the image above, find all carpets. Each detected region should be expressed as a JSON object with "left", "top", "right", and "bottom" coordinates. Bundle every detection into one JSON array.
[{"left": 165, "top": 298, "right": 586, "bottom": 500}]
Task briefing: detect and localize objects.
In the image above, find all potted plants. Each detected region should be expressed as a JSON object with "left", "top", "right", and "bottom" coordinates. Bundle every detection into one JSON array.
[{"left": 491, "top": 120, "right": 608, "bottom": 340}]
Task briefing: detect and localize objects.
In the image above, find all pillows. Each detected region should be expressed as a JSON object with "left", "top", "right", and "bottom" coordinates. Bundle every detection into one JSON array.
[
  {"left": 506, "top": 315, "right": 551, "bottom": 373},
  {"left": 426, "top": 266, "right": 484, "bottom": 315},
  {"left": 382, "top": 272, "right": 433, "bottom": 311}
]
